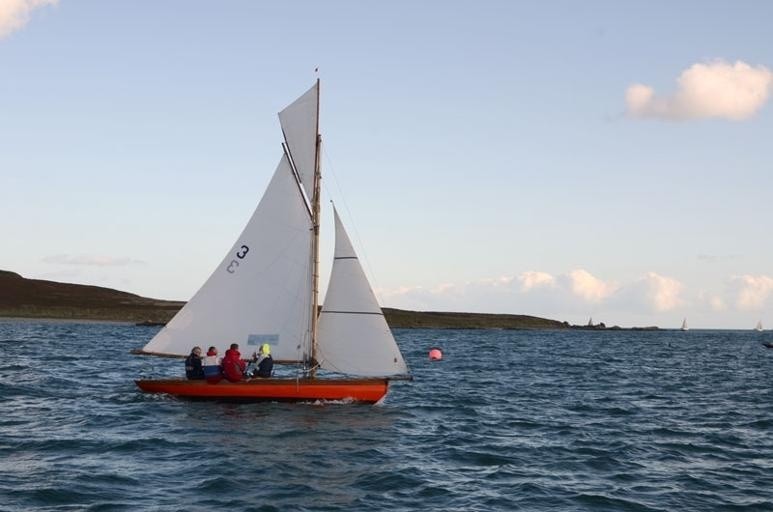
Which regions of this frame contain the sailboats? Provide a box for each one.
[122,73,413,407]
[681,319,687,331]
[588,317,593,326]
[754,320,763,331]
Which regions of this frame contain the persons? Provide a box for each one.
[184,346,205,380]
[223,344,246,383]
[244,343,274,378]
[202,347,224,384]
